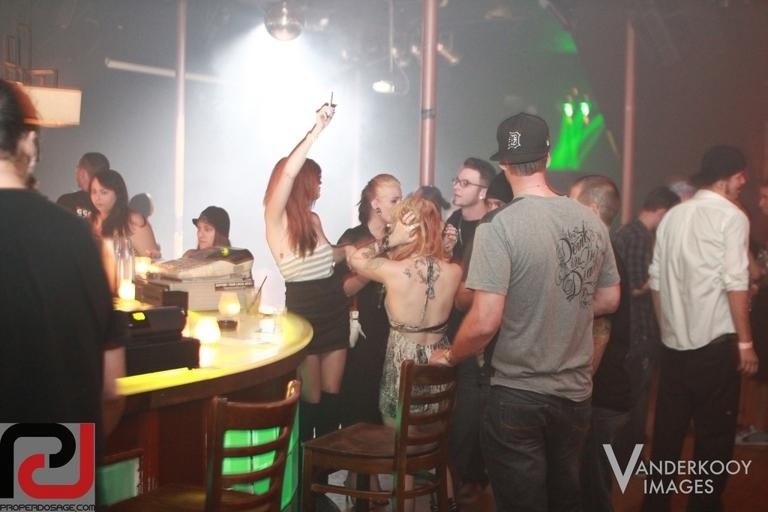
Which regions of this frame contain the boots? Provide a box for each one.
[298,390,341,495]
[430,495,459,512]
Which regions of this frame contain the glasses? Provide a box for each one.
[452,175,489,191]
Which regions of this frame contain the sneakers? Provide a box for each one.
[734,424,768,446]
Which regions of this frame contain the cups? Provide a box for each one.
[161,289,189,319]
[245,288,263,316]
[114,237,137,298]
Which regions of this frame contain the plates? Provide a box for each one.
[217,320,238,330]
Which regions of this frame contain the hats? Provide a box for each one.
[192,205,230,236]
[688,144,747,187]
[489,111,550,164]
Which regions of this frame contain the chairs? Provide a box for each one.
[107,376,305,512]
[306,359,459,511]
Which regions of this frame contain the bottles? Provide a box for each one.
[219,292,243,317]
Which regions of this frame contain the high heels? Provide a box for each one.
[344,478,390,506]
